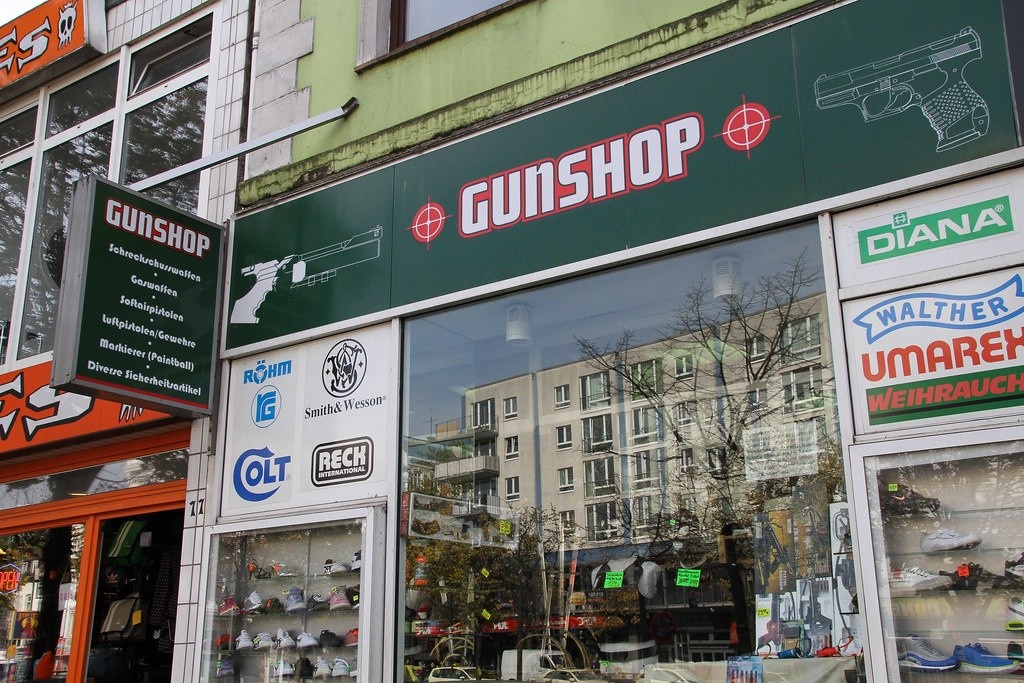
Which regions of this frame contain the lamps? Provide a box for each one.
[505,305,532,346]
[711,257,741,300]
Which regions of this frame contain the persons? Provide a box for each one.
[591,539,661,602]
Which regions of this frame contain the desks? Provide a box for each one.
[644,655,860,683]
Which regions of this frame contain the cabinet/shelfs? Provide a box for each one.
[848,424,1024,683]
[199,505,383,683]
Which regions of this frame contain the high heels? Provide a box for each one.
[244,559,300,581]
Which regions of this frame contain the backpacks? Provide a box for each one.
[88,514,156,683]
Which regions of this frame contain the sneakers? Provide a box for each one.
[219,584,360,615]
[216,654,357,679]
[218,626,358,652]
[1005,596,1024,631]
[885,482,942,515]
[888,553,1024,597]
[919,527,982,555]
[322,550,361,576]
[895,634,1024,675]
